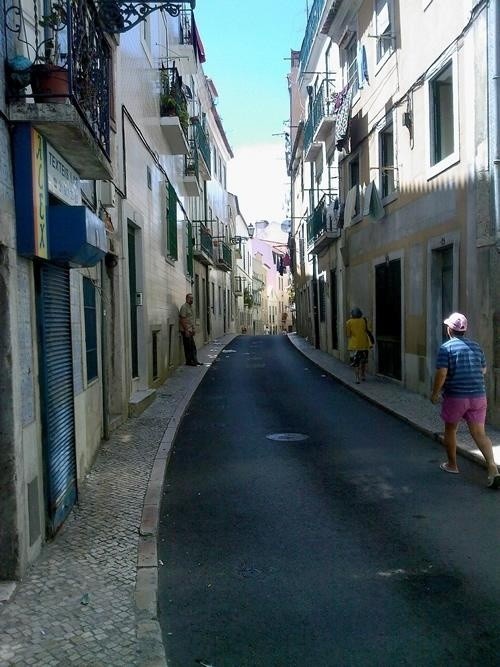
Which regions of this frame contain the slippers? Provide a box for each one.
[439,462,460,474]
[486,473,499,489]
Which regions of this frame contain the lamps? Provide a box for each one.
[240,220,256,240]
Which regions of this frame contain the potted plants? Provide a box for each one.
[161,93,189,128]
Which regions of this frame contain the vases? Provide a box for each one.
[27,63,70,103]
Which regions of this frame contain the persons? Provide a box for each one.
[346,308,372,384]
[179,294,204,366]
[430,312,500,488]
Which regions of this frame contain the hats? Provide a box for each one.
[352,308,361,316]
[443,312,468,332]
